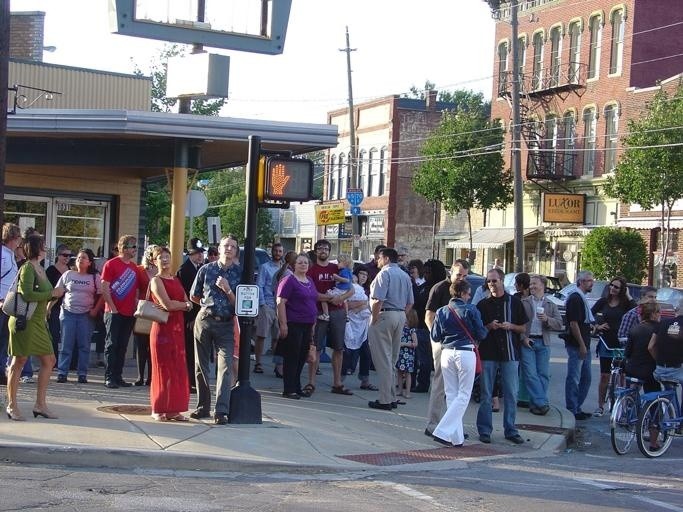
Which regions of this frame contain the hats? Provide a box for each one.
[187,238,206,255]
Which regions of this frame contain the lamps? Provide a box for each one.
[145,180,161,209]
[544,242,553,257]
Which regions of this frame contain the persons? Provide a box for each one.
[1,223,682,452]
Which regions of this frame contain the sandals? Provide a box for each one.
[283,383,315,399]
[331,384,353,395]
[254,363,263,373]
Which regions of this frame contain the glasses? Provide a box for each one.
[61,253,71,257]
[487,280,497,282]
[610,283,619,288]
[127,245,136,248]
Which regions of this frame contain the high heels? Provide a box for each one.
[33,407,58,419]
[6,406,25,421]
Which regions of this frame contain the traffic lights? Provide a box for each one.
[257,155,314,202]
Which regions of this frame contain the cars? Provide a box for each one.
[200,247,271,283]
[466,273,683,331]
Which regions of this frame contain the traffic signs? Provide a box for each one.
[347,192,364,215]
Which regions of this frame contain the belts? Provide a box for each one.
[210,315,222,321]
[451,346,471,350]
[528,335,543,338]
[381,308,406,311]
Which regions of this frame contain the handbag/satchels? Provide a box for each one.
[474,345,481,372]
[133,300,169,334]
[1,292,38,320]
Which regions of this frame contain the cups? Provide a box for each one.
[536,307,543,321]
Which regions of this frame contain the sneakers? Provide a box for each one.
[594,408,605,417]
[20,375,35,384]
[575,412,592,420]
[425,428,524,447]
[57,376,67,383]
[360,383,430,410]
[78,375,87,383]
[152,407,228,424]
[104,378,150,388]
[517,401,549,415]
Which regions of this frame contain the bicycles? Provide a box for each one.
[636,375,683,459]
[595,334,627,409]
[609,373,667,455]
